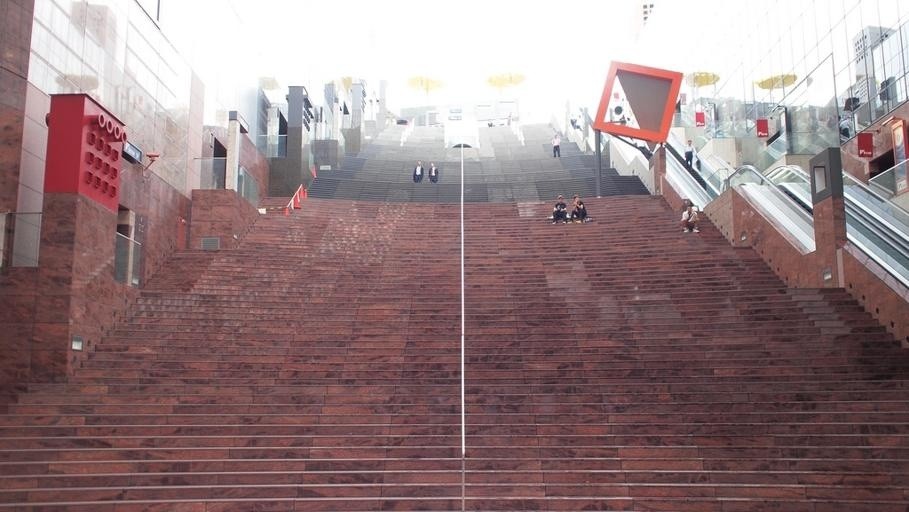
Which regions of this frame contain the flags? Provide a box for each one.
[756,119,768,137]
[695,112,705,126]
[858,133,874,158]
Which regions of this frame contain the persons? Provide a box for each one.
[680,202,700,234]
[412,158,424,184]
[553,194,568,225]
[684,139,694,169]
[551,133,561,158]
[570,194,587,224]
[569,118,583,132]
[428,162,438,183]
